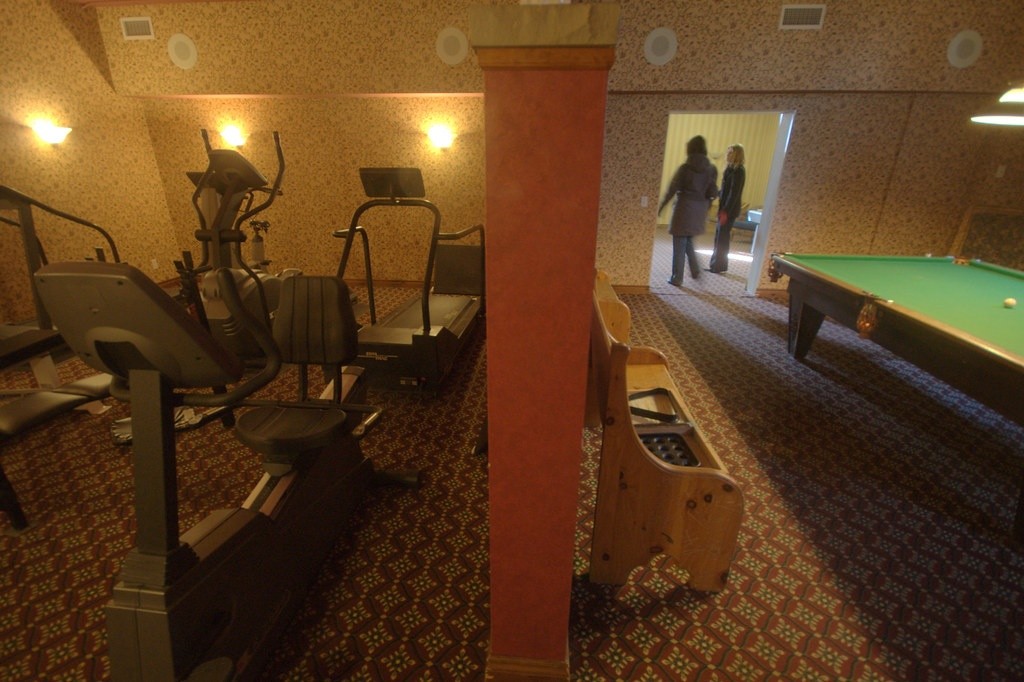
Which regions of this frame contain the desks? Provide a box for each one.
[747,210,762,254]
[766,251,1024,428]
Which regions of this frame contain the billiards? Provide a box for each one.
[862,322,872,333]
[1004,297,1017,308]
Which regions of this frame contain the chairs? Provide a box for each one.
[234,274,360,460]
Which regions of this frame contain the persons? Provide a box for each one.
[658,135,718,286]
[703,143,745,273]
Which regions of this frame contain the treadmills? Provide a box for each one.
[330,166,486,394]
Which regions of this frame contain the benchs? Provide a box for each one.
[585,267,745,594]
[711,218,757,242]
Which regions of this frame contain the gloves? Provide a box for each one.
[718,212,727,225]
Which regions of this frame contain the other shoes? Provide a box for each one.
[692,271,699,278]
[667,279,680,286]
[704,268,719,273]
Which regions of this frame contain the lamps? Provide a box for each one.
[970,81,1024,127]
